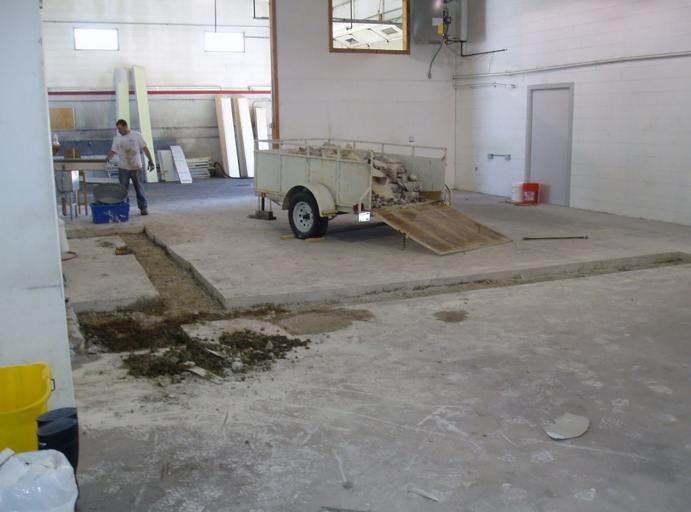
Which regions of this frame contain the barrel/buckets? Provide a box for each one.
[511,182,523,204]
[523,182,539,204]
[55,171,80,192]
[0,361,56,452]
[92,184,129,204]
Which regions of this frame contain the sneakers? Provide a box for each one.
[141,207,147,215]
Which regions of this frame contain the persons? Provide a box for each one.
[105,120,155,215]
